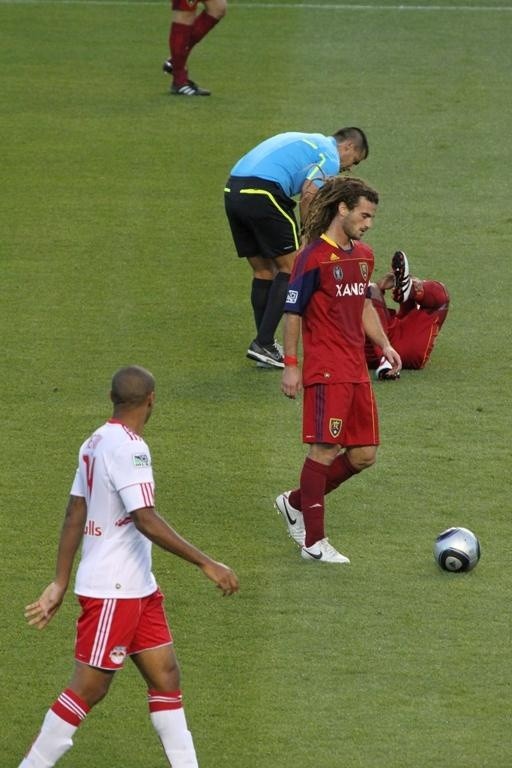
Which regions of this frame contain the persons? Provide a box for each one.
[221,124,373,373]
[162,0,230,97]
[363,247,451,384]
[269,178,406,568]
[10,358,243,768]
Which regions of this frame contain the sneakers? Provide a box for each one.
[164,57,189,76]
[257,342,284,369]
[376,356,401,381]
[247,338,286,368]
[391,250,413,304]
[274,490,308,550]
[171,80,211,96]
[303,538,351,564]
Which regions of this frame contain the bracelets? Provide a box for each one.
[282,352,300,368]
[300,222,311,235]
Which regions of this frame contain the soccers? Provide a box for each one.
[434,527,480,574]
